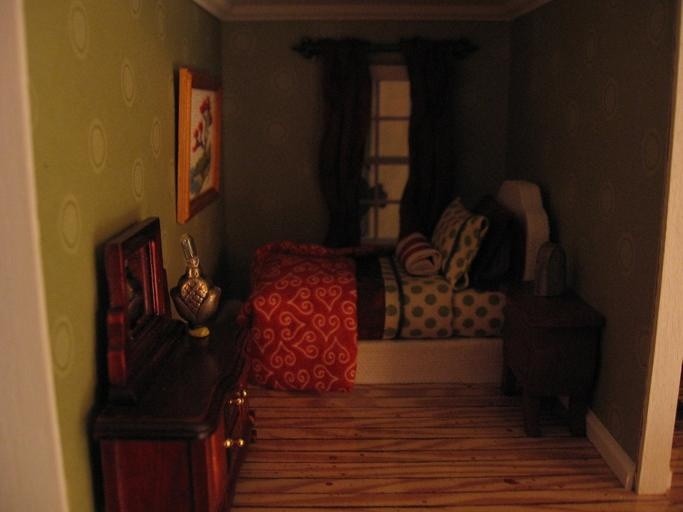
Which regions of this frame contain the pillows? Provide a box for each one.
[395,194,523,293]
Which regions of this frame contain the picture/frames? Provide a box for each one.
[175,65,224,225]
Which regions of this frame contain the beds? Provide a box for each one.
[251,176,552,389]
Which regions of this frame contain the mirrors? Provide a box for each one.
[100,215,184,405]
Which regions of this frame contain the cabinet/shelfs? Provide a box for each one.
[94,327,256,512]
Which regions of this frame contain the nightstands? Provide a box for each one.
[502,284,606,439]
